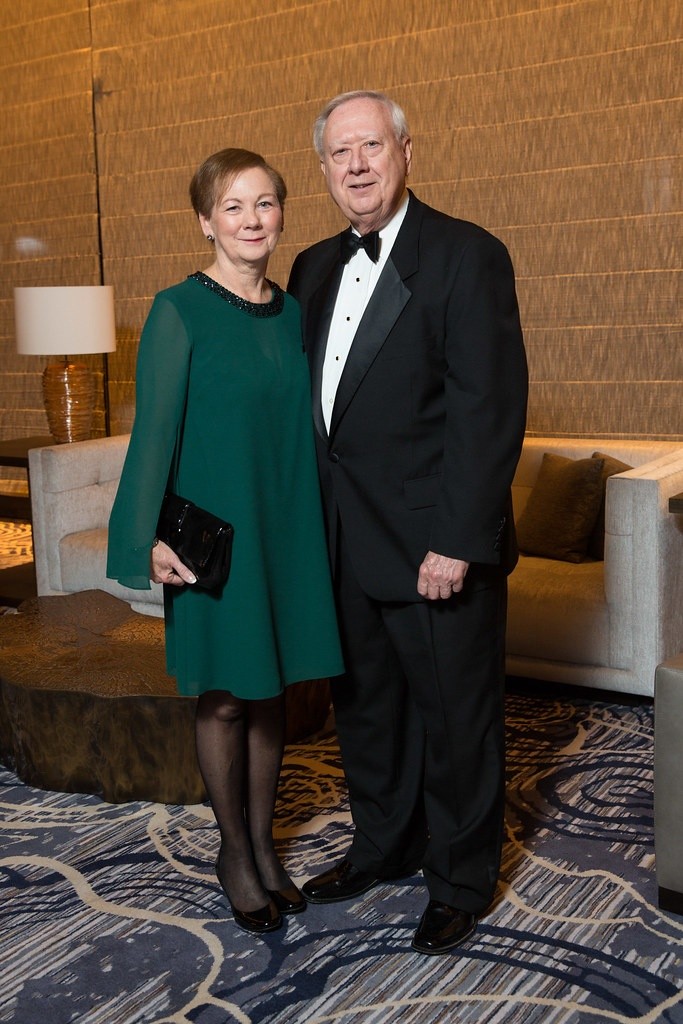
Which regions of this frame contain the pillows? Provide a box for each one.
[589,452,633,562]
[514,450,606,564]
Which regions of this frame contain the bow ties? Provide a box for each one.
[341,231,379,263]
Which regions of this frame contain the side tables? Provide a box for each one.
[0,435,58,609]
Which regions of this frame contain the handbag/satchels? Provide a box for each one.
[156,490,233,598]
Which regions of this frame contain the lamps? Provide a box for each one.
[13,285,118,444]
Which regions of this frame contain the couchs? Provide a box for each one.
[28,433,683,708]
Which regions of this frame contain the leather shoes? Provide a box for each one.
[411,900,476,954]
[301,860,418,903]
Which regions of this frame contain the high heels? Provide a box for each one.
[257,864,306,914]
[215,845,283,932]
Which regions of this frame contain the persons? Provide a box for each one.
[105,148,345,931]
[286,91,529,955]
